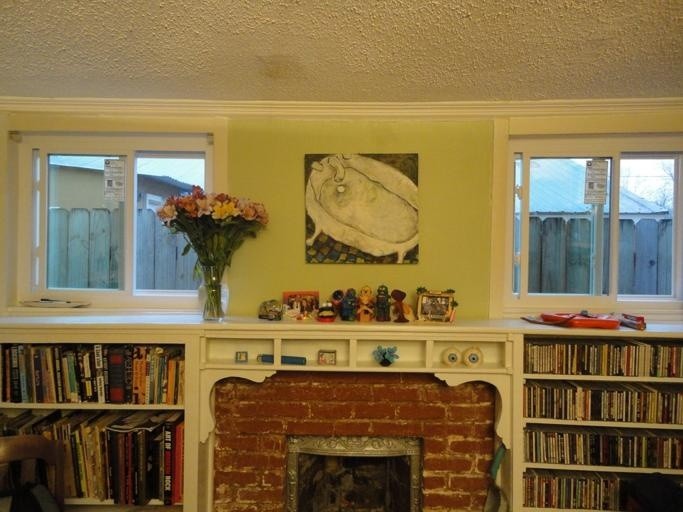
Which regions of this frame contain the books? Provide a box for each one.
[524,340,683,509]
[1,343,186,404]
[0,412,184,506]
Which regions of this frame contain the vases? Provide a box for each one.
[202,263,224,320]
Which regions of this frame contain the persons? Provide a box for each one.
[290,294,311,314]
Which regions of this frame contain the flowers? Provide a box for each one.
[156,184,269,318]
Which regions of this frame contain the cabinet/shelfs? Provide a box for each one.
[200,327,512,375]
[512,335,683,512]
[0,327,201,511]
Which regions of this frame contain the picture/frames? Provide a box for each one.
[418,293,453,321]
[282,290,320,319]
